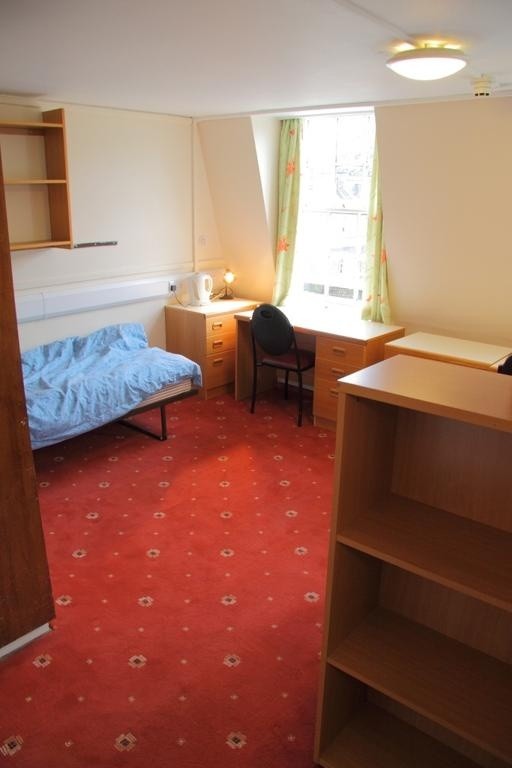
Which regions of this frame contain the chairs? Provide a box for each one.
[250,304,314,427]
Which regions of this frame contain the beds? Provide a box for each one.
[20,322,202,451]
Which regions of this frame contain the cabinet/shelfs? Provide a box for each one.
[0,107,74,251]
[313,354,512,768]
[166,296,263,400]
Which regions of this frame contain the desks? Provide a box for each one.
[234,313,404,434]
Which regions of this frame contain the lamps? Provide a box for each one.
[385,38,468,82]
[218,269,235,299]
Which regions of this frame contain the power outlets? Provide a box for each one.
[169,281,177,293]
